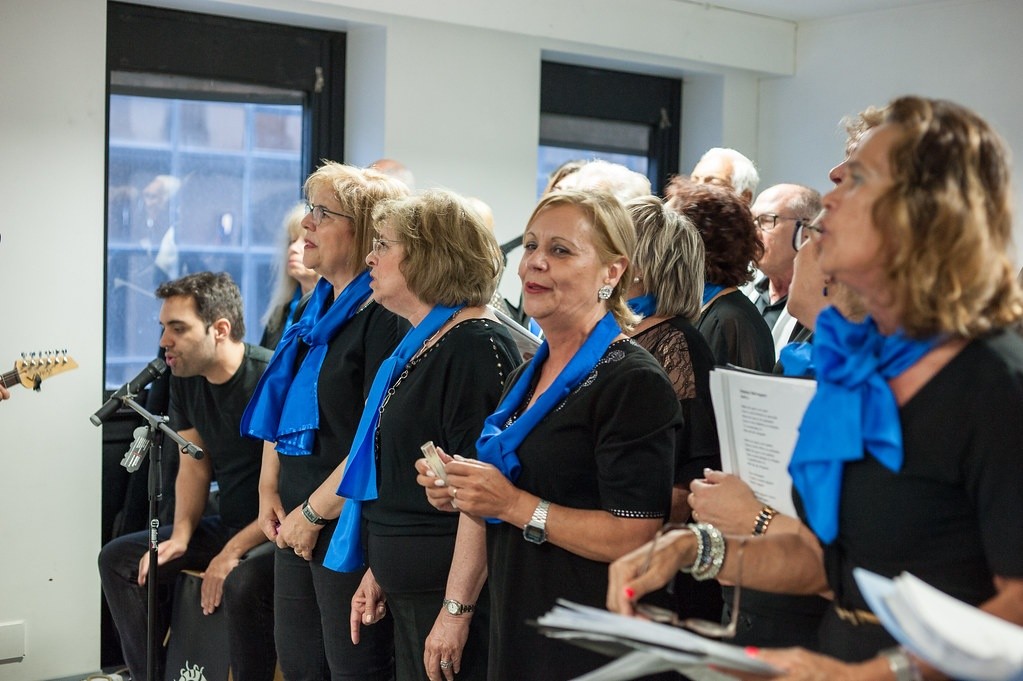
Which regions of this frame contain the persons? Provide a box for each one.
[98,97,1023,681]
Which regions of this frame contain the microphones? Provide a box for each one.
[90,358,167,427]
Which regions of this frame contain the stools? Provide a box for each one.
[165,568,285,681]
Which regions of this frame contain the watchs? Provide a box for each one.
[523,499,551,545]
[301,499,333,523]
[442,598,477,615]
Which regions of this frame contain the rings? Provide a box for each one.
[440,661,452,669]
[691,511,697,521]
[452,487,458,500]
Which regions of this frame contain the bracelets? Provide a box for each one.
[680,522,726,582]
[881,647,919,681]
[752,506,780,537]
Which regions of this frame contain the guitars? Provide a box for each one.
[1,347,80,394]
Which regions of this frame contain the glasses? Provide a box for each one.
[752,214,810,230]
[636,523,748,638]
[792,221,822,252]
[372,237,402,255]
[305,204,354,226]
